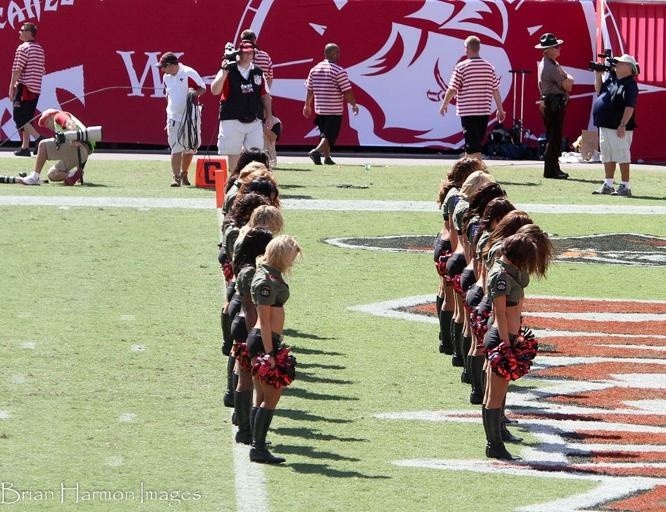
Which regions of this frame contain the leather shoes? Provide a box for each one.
[545,171,567,179]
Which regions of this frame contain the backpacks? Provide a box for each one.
[487,123,512,160]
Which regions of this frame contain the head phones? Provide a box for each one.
[238,40,258,60]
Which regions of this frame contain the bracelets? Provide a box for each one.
[351,102,356,107]
[619,122,627,128]
[220,67,227,72]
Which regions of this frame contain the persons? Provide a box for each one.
[153,48,208,191]
[298,40,361,167]
[7,20,47,157]
[236,28,276,94]
[17,106,96,188]
[214,143,305,466]
[438,34,509,160]
[428,150,555,466]
[590,53,638,199]
[201,38,274,177]
[534,31,575,182]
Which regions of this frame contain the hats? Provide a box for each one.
[614,53,640,75]
[535,33,564,49]
[240,40,254,52]
[154,52,178,68]
[37,109,60,127]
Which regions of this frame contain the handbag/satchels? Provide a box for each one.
[501,143,529,159]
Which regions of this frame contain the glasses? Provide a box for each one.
[244,51,255,55]
[19,29,28,32]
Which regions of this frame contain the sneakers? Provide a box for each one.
[179,171,190,185]
[170,174,181,187]
[592,182,616,195]
[324,159,335,165]
[20,174,40,186]
[308,148,322,165]
[64,169,84,185]
[14,148,31,157]
[611,183,632,198]
[33,136,45,154]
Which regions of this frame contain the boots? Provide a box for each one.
[502,398,518,425]
[502,419,524,443]
[250,407,286,464]
[435,295,487,405]
[485,407,523,461]
[221,306,255,445]
